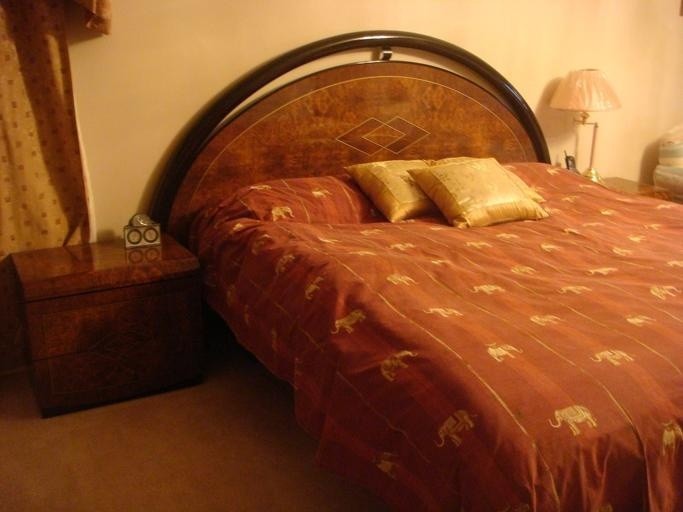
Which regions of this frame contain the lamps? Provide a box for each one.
[549,68,623,187]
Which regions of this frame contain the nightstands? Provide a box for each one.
[10,231,209,420]
[601,177,673,202]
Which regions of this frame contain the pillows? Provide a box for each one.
[208,176,382,226]
[405,158,549,230]
[429,154,544,224]
[345,156,434,222]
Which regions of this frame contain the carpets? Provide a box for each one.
[0,370,392,511]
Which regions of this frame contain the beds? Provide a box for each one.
[149,31,682,511]
